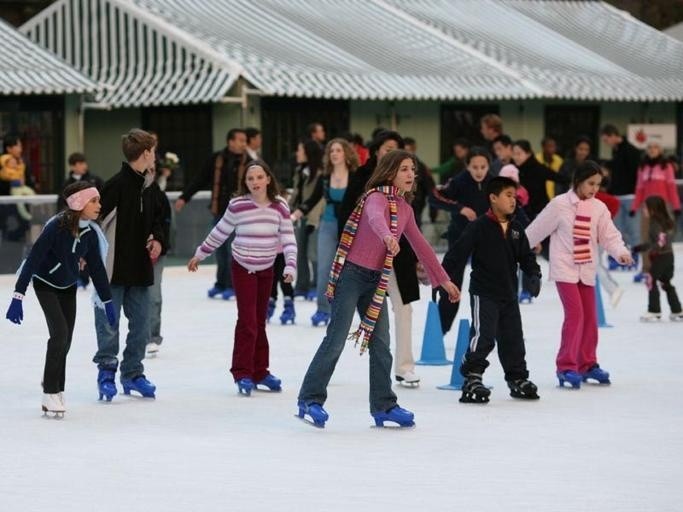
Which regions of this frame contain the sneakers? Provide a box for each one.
[41,393,66,414]
[119,371,158,394]
[296,399,328,424]
[147,342,159,352]
[371,405,415,424]
[607,253,682,321]
[207,284,332,392]
[458,364,611,399]
[96,366,117,396]
[393,370,422,384]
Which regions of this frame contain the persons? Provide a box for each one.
[523,157,637,390]
[77,128,171,401]
[296,148,461,429]
[174,112,682,322]
[382,257,426,391]
[5,181,117,419]
[0,136,108,211]
[434,177,541,404]
[186,160,298,398]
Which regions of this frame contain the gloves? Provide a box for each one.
[5,292,25,326]
[103,299,117,330]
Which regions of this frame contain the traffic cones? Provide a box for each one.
[437,318,492,392]
[594,272,612,328]
[416,301,453,366]
